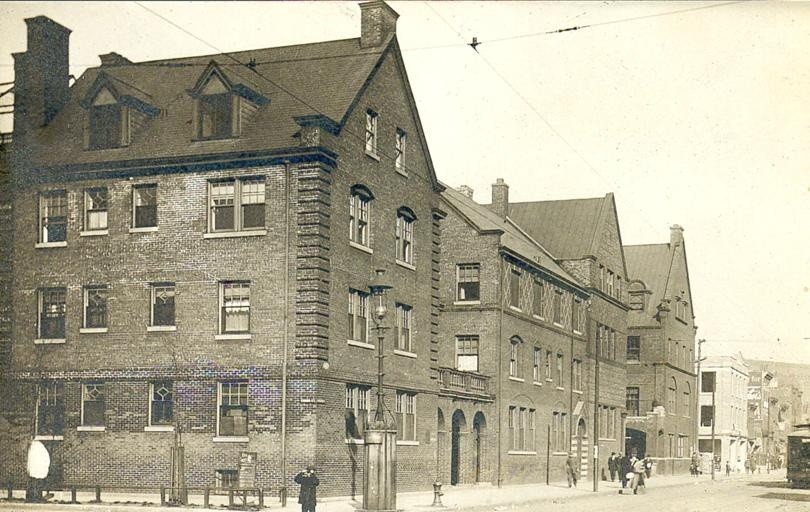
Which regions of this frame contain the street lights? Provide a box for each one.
[367,268,396,428]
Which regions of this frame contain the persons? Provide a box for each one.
[691,451,756,476]
[608,453,653,488]
[294,467,319,511]
